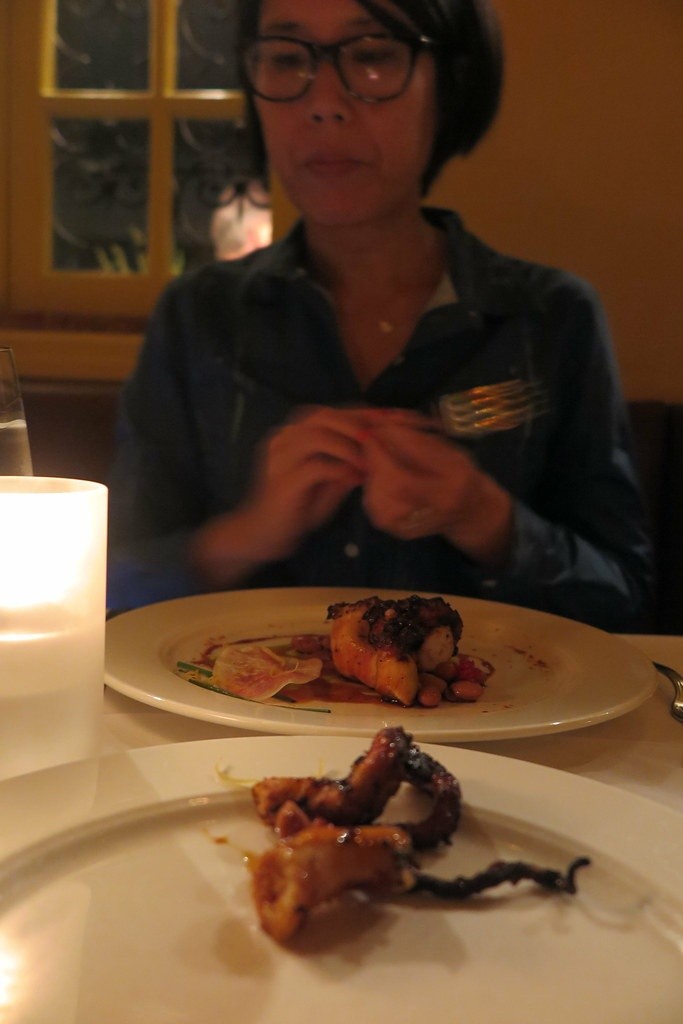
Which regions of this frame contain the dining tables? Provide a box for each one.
[106,635,683,811]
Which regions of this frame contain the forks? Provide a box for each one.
[425,380,548,438]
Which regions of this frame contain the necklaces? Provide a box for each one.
[308,229,441,334]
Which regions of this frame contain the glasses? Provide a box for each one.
[236,32,434,104]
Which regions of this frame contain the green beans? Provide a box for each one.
[419,661,483,706]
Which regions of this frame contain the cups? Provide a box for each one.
[0,346,35,476]
[0,476,108,699]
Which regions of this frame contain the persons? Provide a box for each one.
[107,0,670,635]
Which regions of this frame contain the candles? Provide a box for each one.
[0,477,108,776]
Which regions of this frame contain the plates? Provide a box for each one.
[0,737,683,1024]
[106,586,658,735]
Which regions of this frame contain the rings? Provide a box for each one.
[402,500,430,526]
